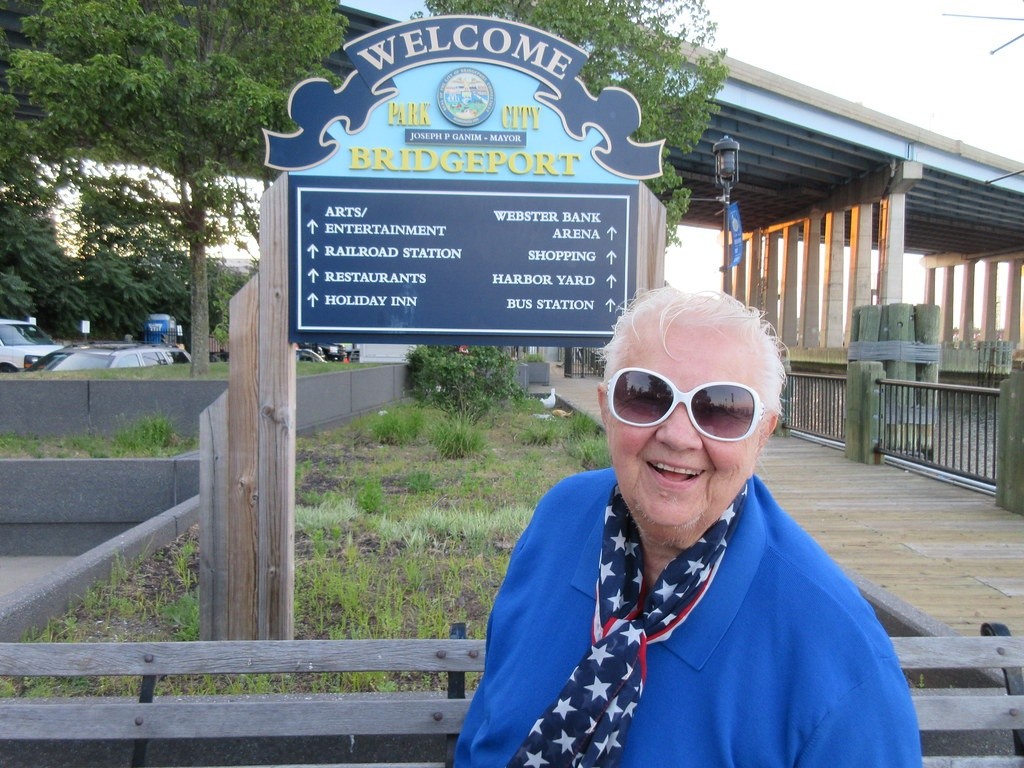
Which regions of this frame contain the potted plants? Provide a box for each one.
[525,353,550,383]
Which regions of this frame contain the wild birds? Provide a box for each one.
[540,388,574,417]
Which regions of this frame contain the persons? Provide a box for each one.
[453,287,924,768]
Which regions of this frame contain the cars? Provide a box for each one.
[0,318,67,373]
[19,339,192,372]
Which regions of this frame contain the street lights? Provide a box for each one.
[710,134,741,295]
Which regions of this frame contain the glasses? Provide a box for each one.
[608,367,769,442]
